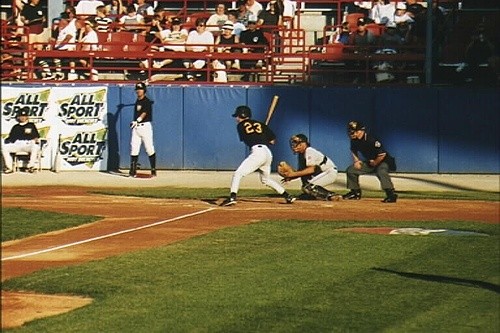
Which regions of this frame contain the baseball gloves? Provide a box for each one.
[276,160,296,179]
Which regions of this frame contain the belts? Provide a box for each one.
[137,124,144,126]
[258,146,262,148]
[334,168,337,169]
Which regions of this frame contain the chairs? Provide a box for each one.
[15,137,48,172]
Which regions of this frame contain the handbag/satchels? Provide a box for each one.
[385,157,396,171]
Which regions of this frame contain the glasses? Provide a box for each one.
[196,24,204,27]
[248,22,255,24]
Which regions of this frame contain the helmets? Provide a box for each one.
[232,106,251,118]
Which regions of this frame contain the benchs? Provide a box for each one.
[0,0,500,83]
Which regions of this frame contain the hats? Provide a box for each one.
[172,17,181,25]
[222,15,258,30]
[357,2,406,27]
[295,134,310,147]
[347,120,360,135]
[135,82,145,90]
[19,109,28,116]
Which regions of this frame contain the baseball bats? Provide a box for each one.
[53,133,63,173]
[264,95,279,126]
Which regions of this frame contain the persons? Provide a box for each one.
[342,122,396,203]
[0,0,500,177]
[220,107,296,206]
[278,134,343,201]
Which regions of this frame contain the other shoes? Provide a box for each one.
[151,170,156,177]
[231,61,262,68]
[343,191,361,200]
[384,194,397,202]
[221,197,237,205]
[42,72,99,82]
[129,173,136,178]
[299,194,316,200]
[5,169,12,173]
[29,167,34,173]
[329,194,343,201]
[286,195,296,204]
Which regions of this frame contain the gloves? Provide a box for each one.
[130,120,139,129]
